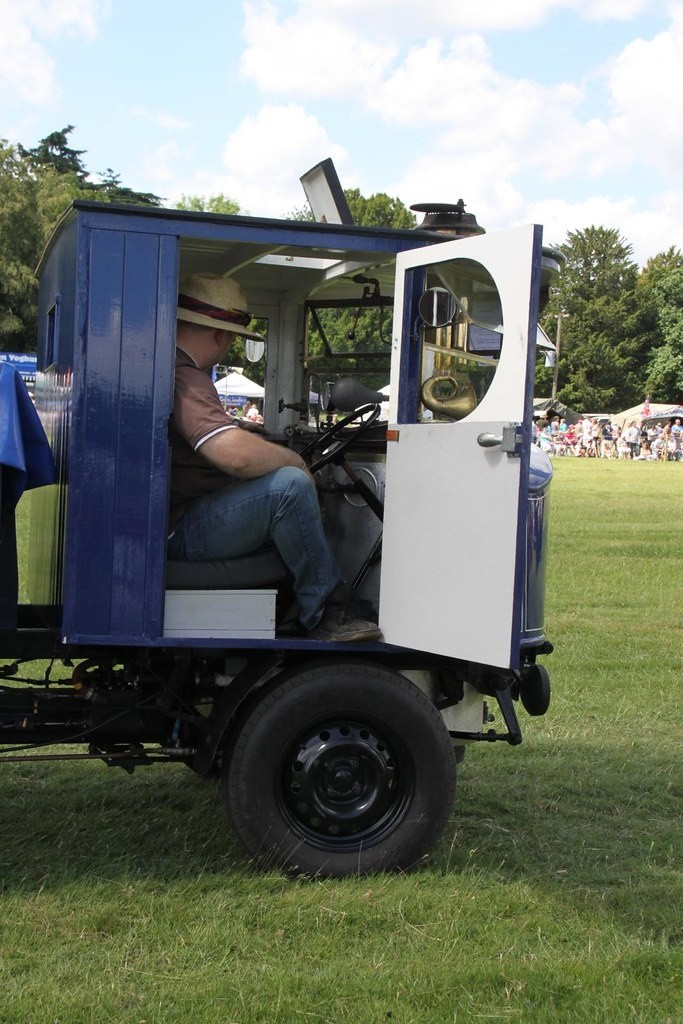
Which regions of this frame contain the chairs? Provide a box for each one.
[160,547,288,587]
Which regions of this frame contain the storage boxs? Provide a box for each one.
[165,588,279,639]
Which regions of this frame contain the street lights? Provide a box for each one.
[550,308,571,400]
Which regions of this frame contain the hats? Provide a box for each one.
[177,271,265,342]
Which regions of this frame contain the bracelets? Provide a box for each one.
[301,462,305,471]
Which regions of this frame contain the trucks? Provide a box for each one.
[0,157,566,882]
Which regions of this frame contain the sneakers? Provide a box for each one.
[315,604,382,642]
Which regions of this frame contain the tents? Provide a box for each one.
[610,402,683,435]
[532,398,585,426]
[213,372,265,416]
[355,385,433,422]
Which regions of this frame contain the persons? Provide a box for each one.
[166,273,382,644]
[225,401,263,424]
[530,416,683,463]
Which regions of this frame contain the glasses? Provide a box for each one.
[231,306,255,327]
